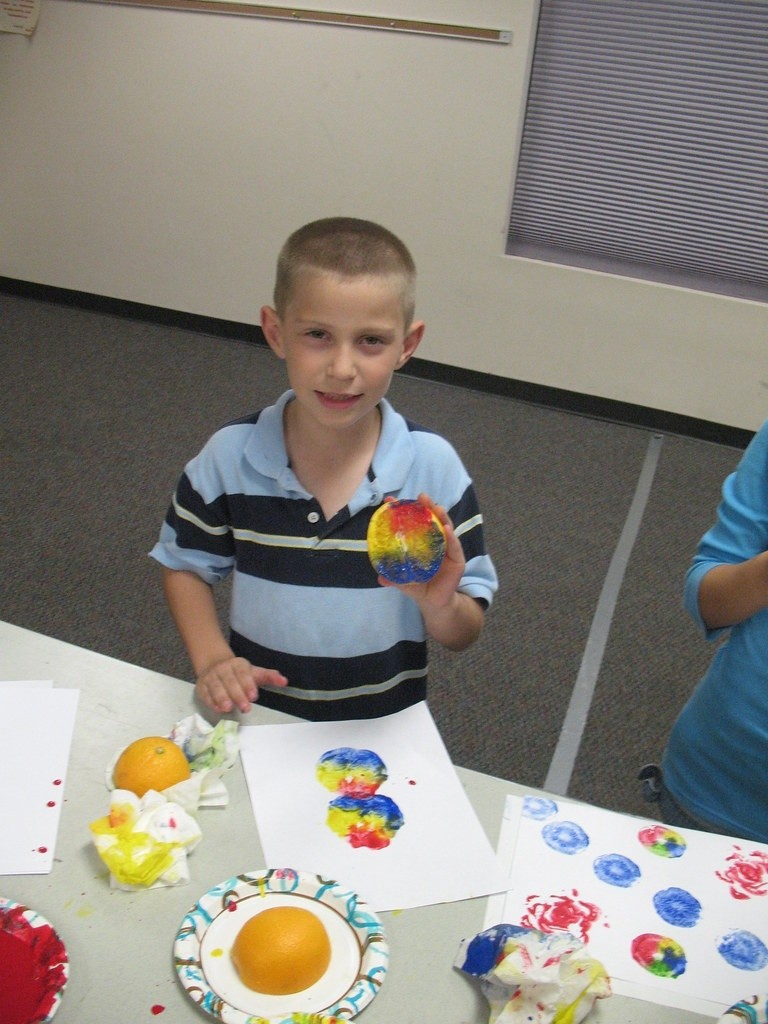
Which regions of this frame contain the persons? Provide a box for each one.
[148,216,499,726]
[634,420,768,844]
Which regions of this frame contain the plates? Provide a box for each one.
[0,896,68,1024]
[173,865,390,1024]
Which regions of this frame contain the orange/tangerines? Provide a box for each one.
[366,498,446,585]
[111,736,189,797]
[230,905,331,995]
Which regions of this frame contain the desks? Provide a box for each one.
[0,623,768,1024]
